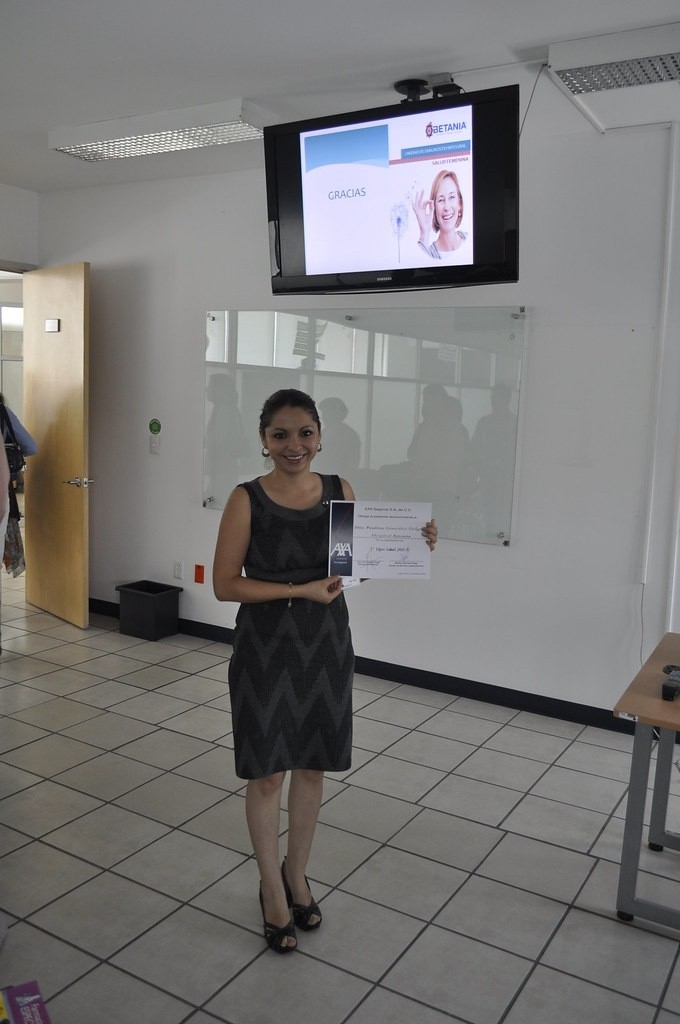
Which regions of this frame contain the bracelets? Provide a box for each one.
[288,582,292,608]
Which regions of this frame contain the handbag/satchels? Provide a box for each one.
[5,446,24,473]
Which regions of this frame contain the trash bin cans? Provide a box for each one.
[116,579,184,642]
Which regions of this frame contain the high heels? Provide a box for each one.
[282,856,323,931]
[259,880,298,953]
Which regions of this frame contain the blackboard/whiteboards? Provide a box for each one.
[199,304,528,548]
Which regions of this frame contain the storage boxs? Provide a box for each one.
[115,579,184,641]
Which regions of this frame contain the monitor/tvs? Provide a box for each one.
[263,85,521,296]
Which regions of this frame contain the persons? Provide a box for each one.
[213,388,437,954]
[0,393,37,657]
[411,170,471,259]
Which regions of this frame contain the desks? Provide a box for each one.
[613,632,680,931]
